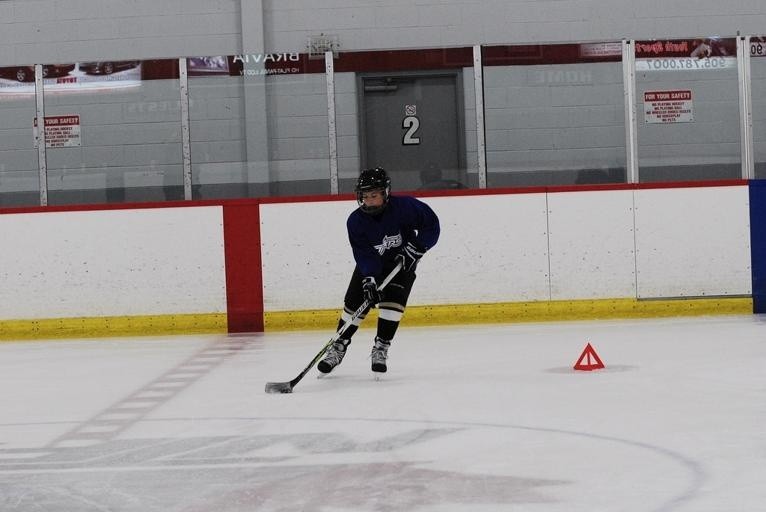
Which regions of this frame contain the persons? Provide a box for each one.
[315,166,442,374]
[416,167,472,192]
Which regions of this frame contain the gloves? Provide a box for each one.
[362,276,384,309]
[394,242,425,273]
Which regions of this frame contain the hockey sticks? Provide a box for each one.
[265,261,403,393]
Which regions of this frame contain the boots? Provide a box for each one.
[372,336,390,373]
[318,338,351,373]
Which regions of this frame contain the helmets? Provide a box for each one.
[419,167,443,185]
[353,167,391,214]
